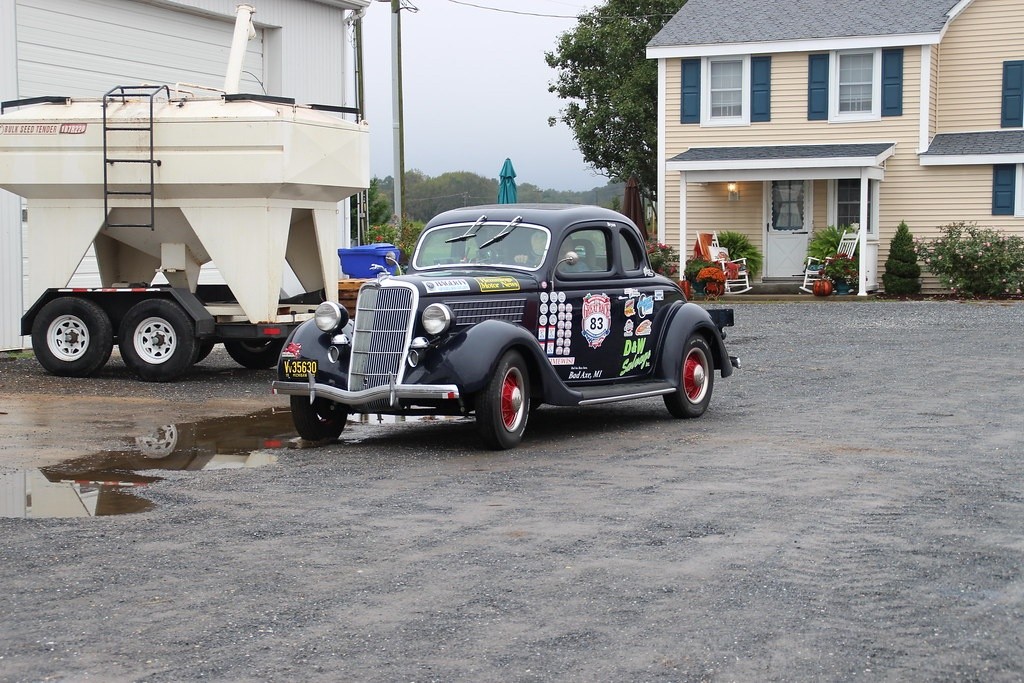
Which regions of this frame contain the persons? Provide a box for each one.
[515,236,589,271]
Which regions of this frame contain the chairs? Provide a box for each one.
[572,238,598,270]
[695,230,753,294]
[799,229,860,294]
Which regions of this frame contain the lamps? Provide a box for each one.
[727,181,737,192]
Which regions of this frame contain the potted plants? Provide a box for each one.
[685,255,717,292]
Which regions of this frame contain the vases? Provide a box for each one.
[812,281,832,296]
[703,279,720,298]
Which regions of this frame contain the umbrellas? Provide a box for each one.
[497,158,518,203]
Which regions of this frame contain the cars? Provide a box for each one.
[273,203,741,451]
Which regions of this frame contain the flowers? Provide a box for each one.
[696,267,726,285]
[818,253,859,291]
[644,237,680,277]
